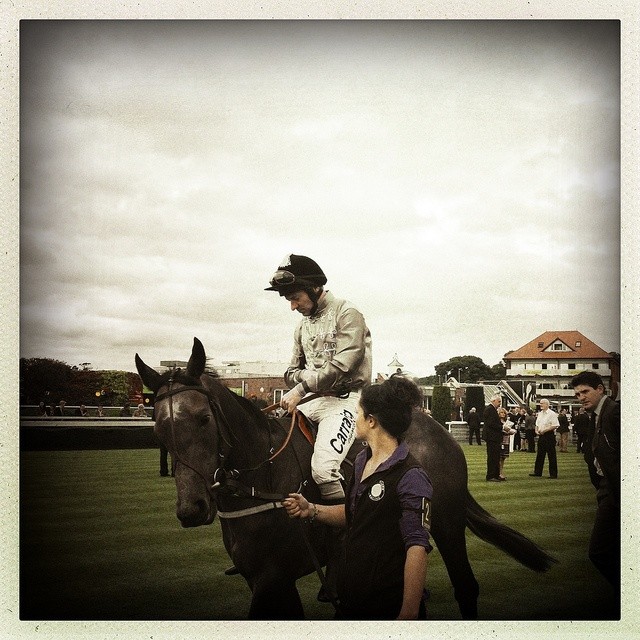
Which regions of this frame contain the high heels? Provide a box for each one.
[523,449,527,452]
[519,449,523,451]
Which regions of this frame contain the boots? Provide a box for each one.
[225,566,241,575]
[318,497,349,603]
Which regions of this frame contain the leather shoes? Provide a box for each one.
[547,477,557,479]
[529,473,542,476]
[497,476,505,481]
[487,478,500,482]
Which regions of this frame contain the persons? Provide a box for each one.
[120,402,132,417]
[134,404,147,417]
[49,403,56,416]
[482,395,505,482]
[466,407,481,445]
[529,398,561,479]
[34,401,50,416]
[571,370,620,594]
[263,254,372,536]
[557,408,589,453]
[54,401,66,416]
[498,407,535,478]
[281,370,434,620]
[76,404,89,417]
[94,404,106,416]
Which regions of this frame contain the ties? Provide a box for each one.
[588,412,598,490]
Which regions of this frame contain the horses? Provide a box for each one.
[134,335,560,621]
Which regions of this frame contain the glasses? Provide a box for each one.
[269,270,325,287]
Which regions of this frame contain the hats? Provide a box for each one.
[264,255,327,291]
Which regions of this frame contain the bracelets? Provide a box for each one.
[305,504,318,524]
